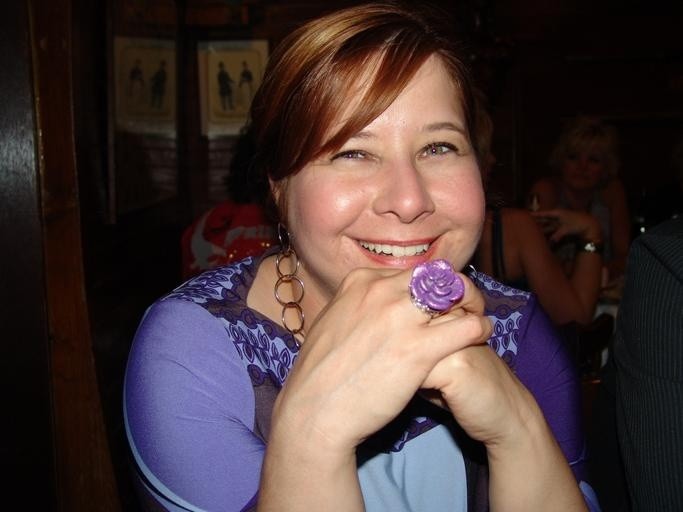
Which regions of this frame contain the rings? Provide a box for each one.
[408,258,465,318]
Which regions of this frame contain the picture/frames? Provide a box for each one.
[113,38,179,134]
[196,39,269,138]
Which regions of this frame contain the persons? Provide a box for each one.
[130,54,170,111]
[124,6,602,512]
[613,214,682,512]
[468,91,606,332]
[217,58,253,111]
[528,112,632,306]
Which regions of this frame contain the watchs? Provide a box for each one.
[574,239,605,255]
